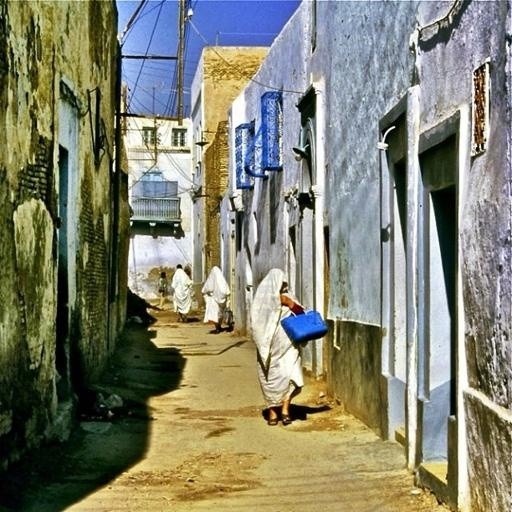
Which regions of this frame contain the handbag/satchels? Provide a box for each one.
[281,310,328,344]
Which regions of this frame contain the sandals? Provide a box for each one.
[281,413,291,425]
[268,417,278,425]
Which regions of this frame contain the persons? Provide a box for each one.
[251,268,306,425]
[159,271,168,307]
[201,265,231,333]
[171,264,193,322]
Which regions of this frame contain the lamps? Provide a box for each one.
[377,126,397,151]
[294,144,310,161]
